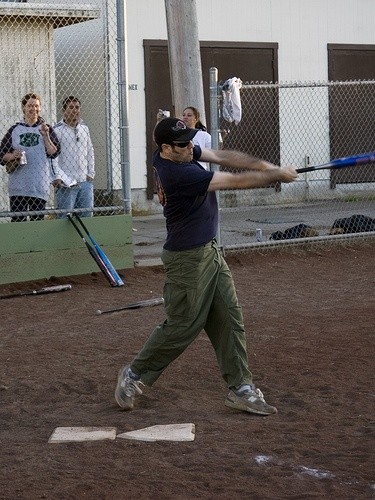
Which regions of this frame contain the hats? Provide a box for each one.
[154,117,199,144]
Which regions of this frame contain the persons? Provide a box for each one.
[157,106,212,172]
[48,96,95,218]
[114,117,299,415]
[0,94,61,223]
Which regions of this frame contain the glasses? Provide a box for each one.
[173,139,192,148]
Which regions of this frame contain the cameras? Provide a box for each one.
[162,111,170,119]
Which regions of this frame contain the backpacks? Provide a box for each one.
[328,215,375,235]
[269,224,319,241]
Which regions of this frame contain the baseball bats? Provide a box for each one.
[96,298,164,316]
[297,152,375,173]
[66,212,124,287]
[0,284,72,297]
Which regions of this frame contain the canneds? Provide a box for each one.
[20,150,28,165]
[256,229,263,242]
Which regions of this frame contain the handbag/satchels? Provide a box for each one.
[6,147,25,175]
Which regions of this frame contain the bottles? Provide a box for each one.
[19,150,27,165]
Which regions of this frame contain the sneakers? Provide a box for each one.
[115,365,145,410]
[225,387,277,416]
